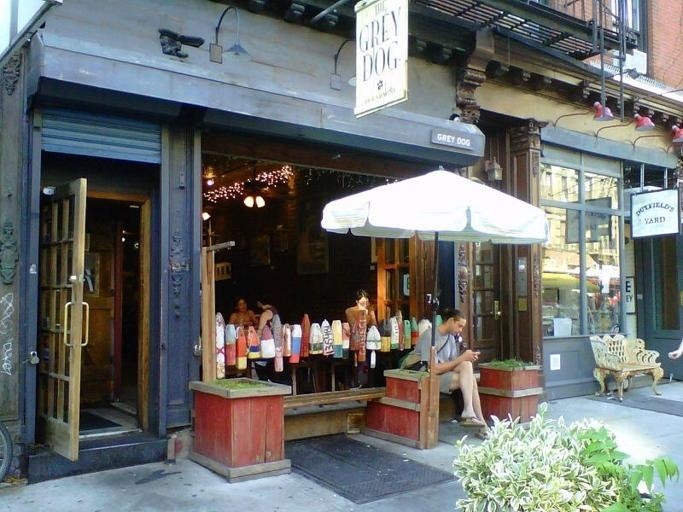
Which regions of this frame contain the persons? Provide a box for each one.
[345,288,378,331]
[254,293,279,338]
[667,338,683,360]
[588,292,618,335]
[228,296,256,329]
[405,308,492,441]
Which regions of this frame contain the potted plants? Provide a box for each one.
[480,358,545,428]
[188,373,294,481]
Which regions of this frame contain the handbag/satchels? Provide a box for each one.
[401,353,426,371]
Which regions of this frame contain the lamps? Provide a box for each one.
[550,98,683,151]
[329,38,358,94]
[483,155,504,182]
[208,4,254,67]
[240,158,268,209]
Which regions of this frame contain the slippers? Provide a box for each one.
[461,417,491,440]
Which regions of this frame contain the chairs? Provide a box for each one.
[590,329,666,402]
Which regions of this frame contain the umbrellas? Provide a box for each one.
[319,169,551,344]
